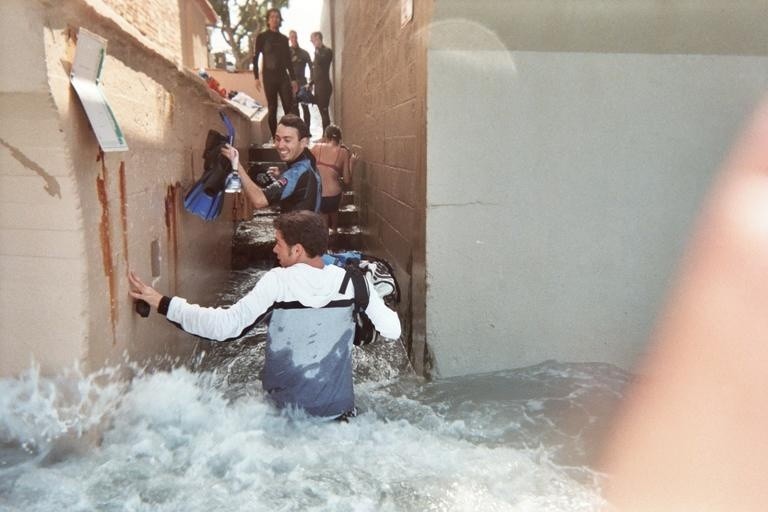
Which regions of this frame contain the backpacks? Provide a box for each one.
[324,249,401,347]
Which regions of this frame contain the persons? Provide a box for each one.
[286,25,313,129]
[127,209,402,424]
[595,86,768,512]
[304,32,333,131]
[221,110,323,268]
[308,124,360,243]
[250,8,299,143]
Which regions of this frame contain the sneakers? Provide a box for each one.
[225,171,242,194]
